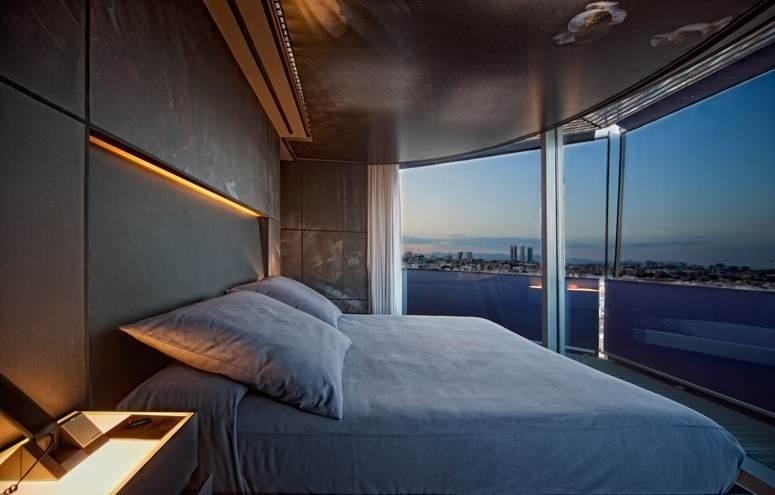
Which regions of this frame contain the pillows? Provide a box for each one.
[118,275,352,423]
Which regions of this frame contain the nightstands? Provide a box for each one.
[0,409,215,493]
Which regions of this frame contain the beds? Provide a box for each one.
[123,315,744,493]
